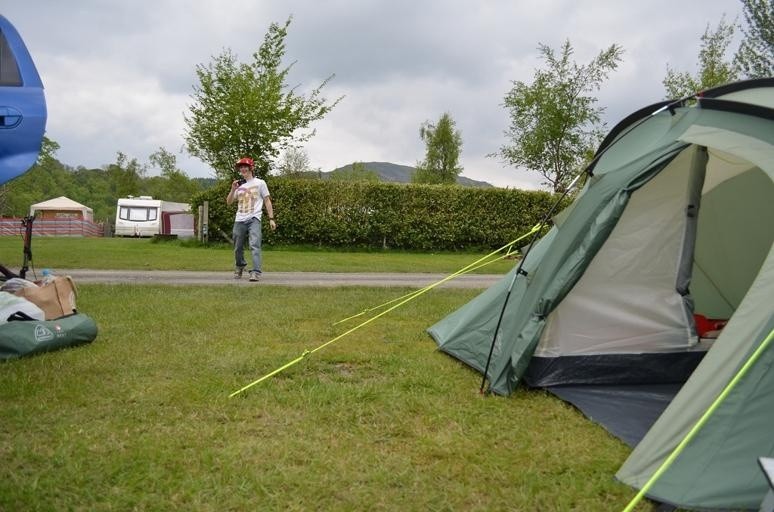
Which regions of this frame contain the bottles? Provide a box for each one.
[41,270,56,287]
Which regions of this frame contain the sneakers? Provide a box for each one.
[248,271,263,281]
[234,266,244,279]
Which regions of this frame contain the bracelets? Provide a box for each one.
[268,218,274,221]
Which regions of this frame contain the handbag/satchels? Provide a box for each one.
[0,277,98,363]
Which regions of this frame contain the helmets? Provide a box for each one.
[235,158,255,167]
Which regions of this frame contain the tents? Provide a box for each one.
[424,80,773,511]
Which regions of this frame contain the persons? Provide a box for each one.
[225,158,276,282]
